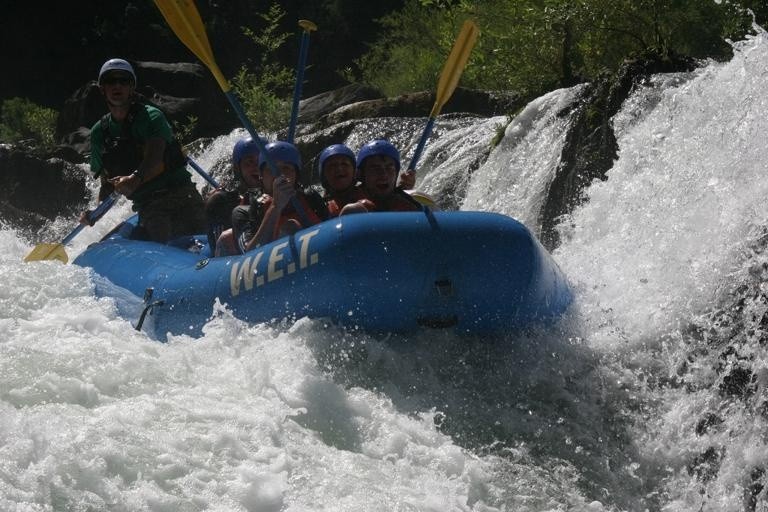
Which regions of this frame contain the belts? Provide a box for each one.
[138,188,180,200]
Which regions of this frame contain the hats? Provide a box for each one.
[98,57,137,91]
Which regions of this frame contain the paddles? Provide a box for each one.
[155,0,313,224]
[24,191,118,263]
[408,20,480,170]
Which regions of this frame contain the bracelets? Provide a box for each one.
[131,170,146,185]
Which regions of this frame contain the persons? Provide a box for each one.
[76,57,208,247]
[230,143,327,250]
[310,144,415,199]
[320,138,423,221]
[203,135,271,259]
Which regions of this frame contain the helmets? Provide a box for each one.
[356,139,400,179]
[232,135,270,166]
[257,141,303,175]
[318,143,357,182]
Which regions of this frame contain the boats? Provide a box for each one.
[73,189,579,343]
